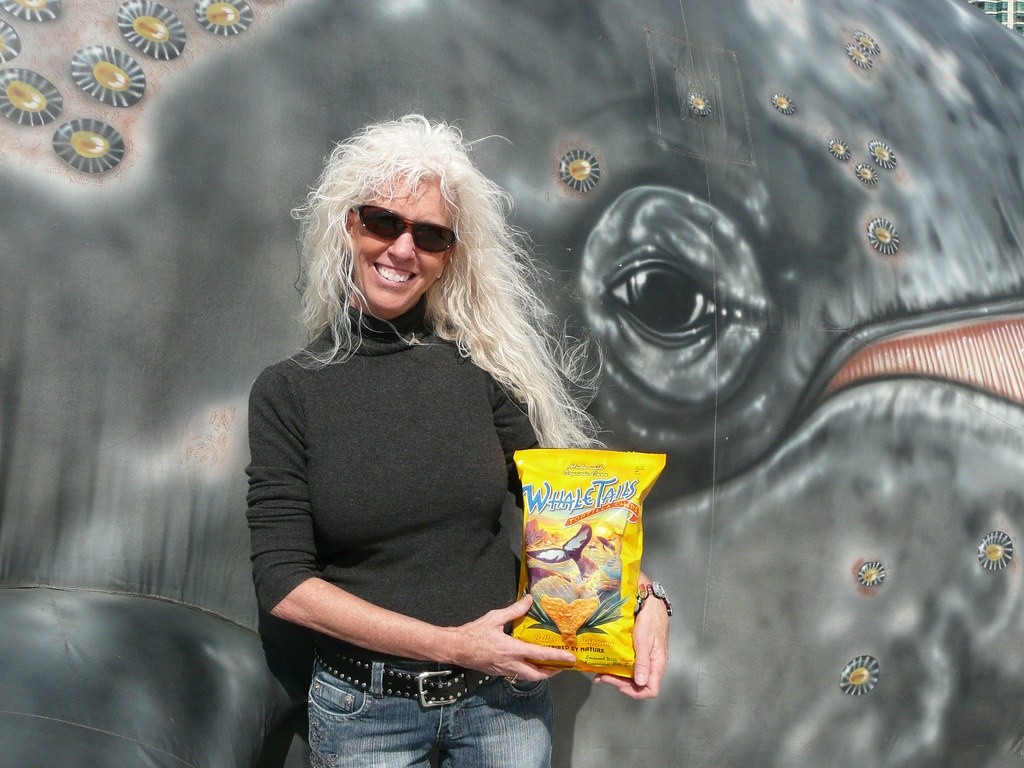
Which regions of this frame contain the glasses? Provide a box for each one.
[353,205,457,255]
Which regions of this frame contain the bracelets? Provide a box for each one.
[634,580,673,618]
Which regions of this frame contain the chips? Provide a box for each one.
[542,595,598,639]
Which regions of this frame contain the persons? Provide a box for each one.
[248,113,674,768]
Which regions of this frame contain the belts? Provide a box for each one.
[309,635,500,707]
